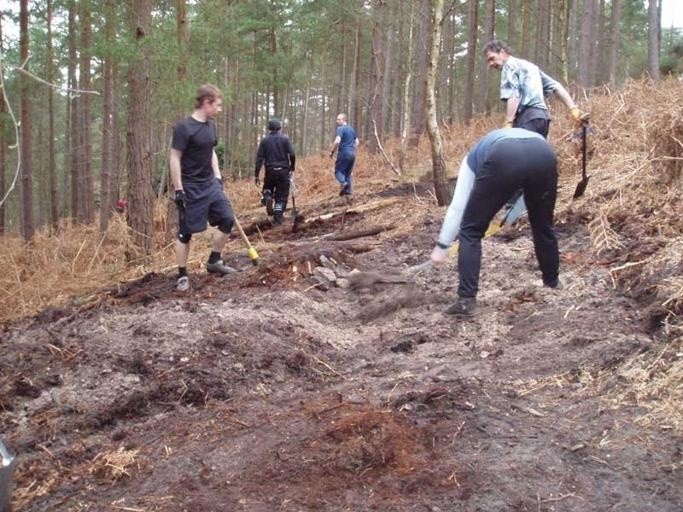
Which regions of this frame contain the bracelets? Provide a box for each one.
[330,149,334,153]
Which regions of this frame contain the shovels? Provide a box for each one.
[573,112,591,198]
[288,196,296,233]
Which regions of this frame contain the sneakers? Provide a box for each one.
[338,182,352,196]
[542,279,565,292]
[439,296,481,318]
[204,259,236,275]
[176,275,190,292]
[264,197,285,225]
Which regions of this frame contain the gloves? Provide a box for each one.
[502,121,513,129]
[215,177,224,192]
[568,104,587,126]
[172,189,190,210]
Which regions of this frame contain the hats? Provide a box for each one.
[267,119,282,132]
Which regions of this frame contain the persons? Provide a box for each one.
[481,38,589,238]
[428,125,563,318]
[254,117,296,224]
[169,83,239,294]
[328,112,360,201]
